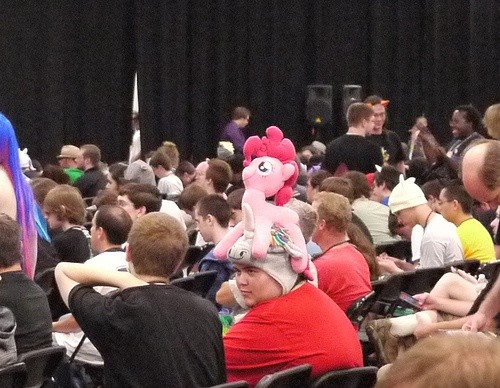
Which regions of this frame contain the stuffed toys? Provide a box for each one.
[213,126,314,281]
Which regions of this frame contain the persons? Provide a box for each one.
[0,95,500,388]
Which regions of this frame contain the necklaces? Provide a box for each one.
[423,210,434,229]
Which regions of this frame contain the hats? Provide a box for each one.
[387,174,428,215]
[55,144,81,159]
[226,202,304,297]
[123,159,156,186]
[18,147,30,168]
[311,140,327,154]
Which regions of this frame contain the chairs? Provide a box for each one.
[0,241,500,388]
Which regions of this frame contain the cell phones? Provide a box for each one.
[398,291,423,310]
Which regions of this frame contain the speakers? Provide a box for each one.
[342,85,362,122]
[305,84,332,126]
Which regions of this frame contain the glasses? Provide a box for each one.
[448,118,466,128]
[115,199,144,207]
[42,209,63,219]
